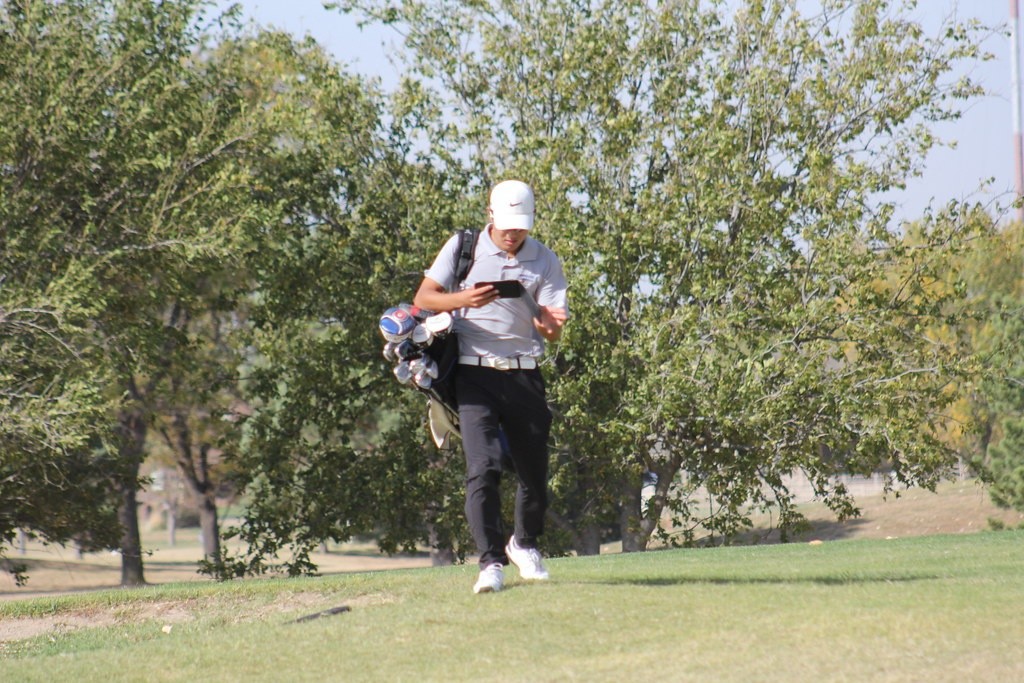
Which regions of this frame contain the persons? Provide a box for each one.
[412,180,568,594]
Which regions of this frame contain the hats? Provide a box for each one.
[489,179,535,231]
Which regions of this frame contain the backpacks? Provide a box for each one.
[395,228,482,396]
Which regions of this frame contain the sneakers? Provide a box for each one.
[472,562,504,594]
[504,534,549,581]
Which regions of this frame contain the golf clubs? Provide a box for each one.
[383,339,439,390]
